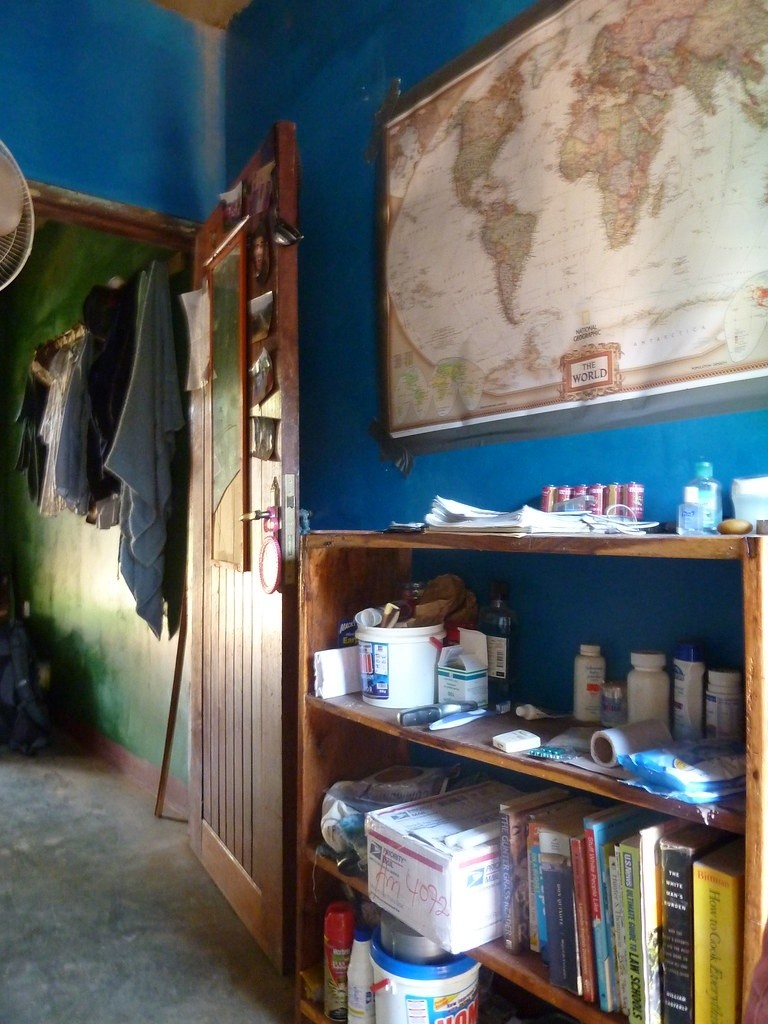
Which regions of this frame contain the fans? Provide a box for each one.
[0,138,36,290]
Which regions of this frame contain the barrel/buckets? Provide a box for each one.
[369,925,483,1023]
[354,624,446,709]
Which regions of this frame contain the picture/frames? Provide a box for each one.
[368,0,768,476]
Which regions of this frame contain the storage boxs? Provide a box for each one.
[365,779,527,953]
[435,627,490,709]
[366,763,444,786]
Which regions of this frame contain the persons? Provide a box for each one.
[249,229,268,284]
[509,812,531,864]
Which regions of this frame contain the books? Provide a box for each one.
[498,788,744,1024]
[425,495,593,534]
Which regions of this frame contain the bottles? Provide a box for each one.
[706,668,742,739]
[678,488,703,535]
[627,653,669,724]
[575,644,605,722]
[684,463,722,531]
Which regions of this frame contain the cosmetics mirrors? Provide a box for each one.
[258,507,281,594]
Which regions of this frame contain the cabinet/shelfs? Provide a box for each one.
[293,531,768,1024]
